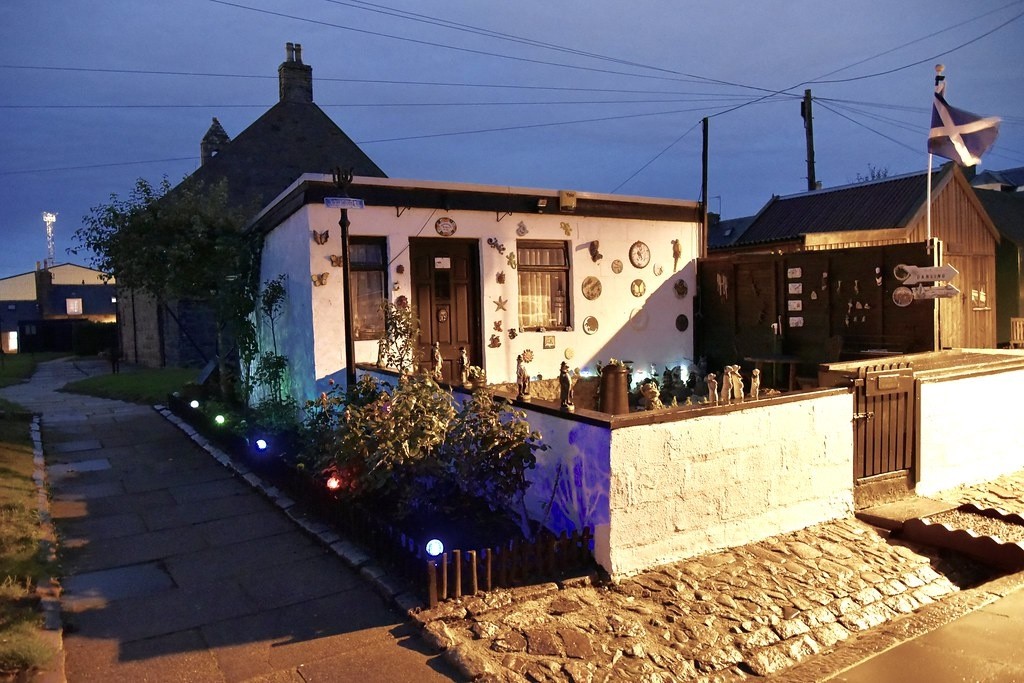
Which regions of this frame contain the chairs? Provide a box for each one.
[794,334,843,389]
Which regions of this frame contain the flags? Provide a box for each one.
[927,77,999,170]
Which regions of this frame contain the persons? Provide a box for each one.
[517,355,530,396]
[707,372,720,406]
[658,395,692,409]
[749,368,761,400]
[459,347,471,383]
[431,342,444,379]
[559,361,574,407]
[720,366,733,405]
[731,364,745,404]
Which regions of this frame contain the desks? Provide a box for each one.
[743,352,802,392]
